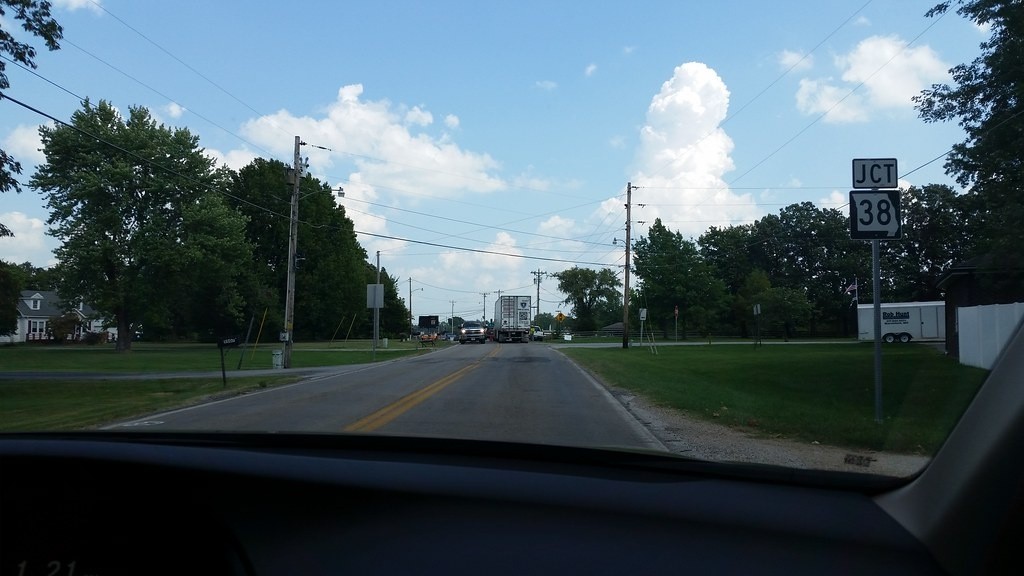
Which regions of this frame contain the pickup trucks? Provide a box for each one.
[530,325,545,342]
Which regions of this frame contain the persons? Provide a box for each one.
[530,325,534,342]
[421,333,435,347]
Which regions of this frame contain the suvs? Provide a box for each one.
[457,320,487,344]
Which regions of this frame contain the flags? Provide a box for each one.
[844,281,856,295]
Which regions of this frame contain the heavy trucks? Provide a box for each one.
[492,295,531,344]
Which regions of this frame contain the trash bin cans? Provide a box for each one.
[383,338,388,349]
[271,350,284,369]
